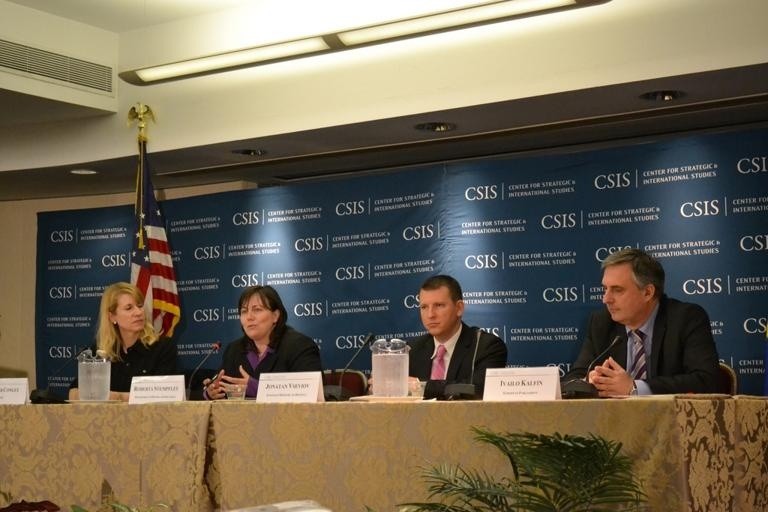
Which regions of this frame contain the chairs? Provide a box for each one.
[322,367,368,402]
[718,362,738,396]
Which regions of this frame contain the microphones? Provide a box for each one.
[188,341,222,391]
[325,332,375,399]
[562,335,622,396]
[444,328,482,397]
[30,347,88,403]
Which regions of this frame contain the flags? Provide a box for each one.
[130,133,181,338]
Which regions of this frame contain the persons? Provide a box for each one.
[368,275,508,400]
[68,282,178,402]
[562,249,719,398]
[202,286,321,401]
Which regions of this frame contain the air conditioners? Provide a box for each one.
[0,39,114,93]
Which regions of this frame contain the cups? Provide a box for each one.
[409,381,427,400]
[226,385,247,401]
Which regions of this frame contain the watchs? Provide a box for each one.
[629,380,638,395]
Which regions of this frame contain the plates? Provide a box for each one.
[64,399,123,404]
[350,395,425,402]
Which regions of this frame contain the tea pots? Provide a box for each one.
[371,339,411,398]
[78,351,110,400]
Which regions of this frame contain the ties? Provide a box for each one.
[628,328,648,380]
[430,344,446,381]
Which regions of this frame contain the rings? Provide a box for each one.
[237,387,241,392]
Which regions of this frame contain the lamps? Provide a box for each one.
[119,36,334,86]
[334,0,612,53]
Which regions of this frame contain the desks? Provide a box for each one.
[0,392,768,512]
